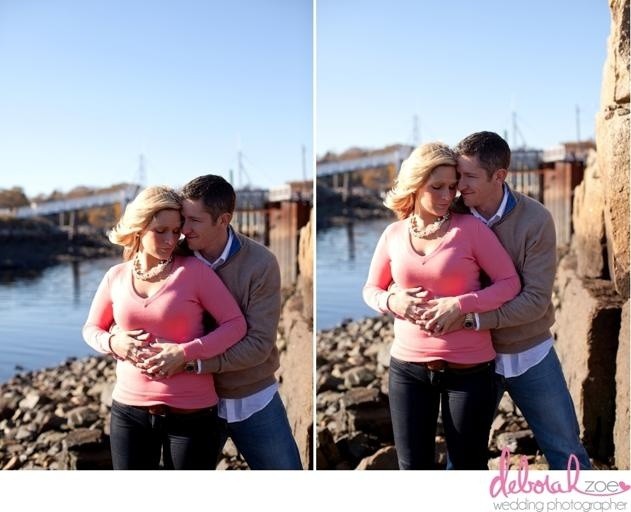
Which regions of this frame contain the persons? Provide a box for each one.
[112,174,303,469]
[82,185,249,469]
[388,131,595,470]
[363,141,524,469]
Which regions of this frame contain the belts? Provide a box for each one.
[416,360,481,372]
[137,405,199,415]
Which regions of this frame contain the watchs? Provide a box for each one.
[184,360,196,373]
[465,313,474,330]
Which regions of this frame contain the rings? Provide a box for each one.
[437,323,443,329]
[159,370,166,376]
[134,349,138,356]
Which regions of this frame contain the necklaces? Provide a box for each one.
[409,210,450,238]
[130,254,173,280]
